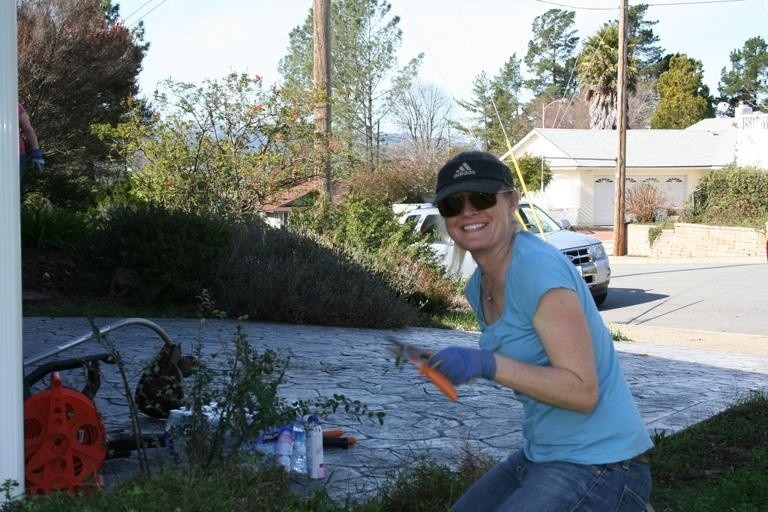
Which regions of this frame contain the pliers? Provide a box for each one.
[383,337,458,401]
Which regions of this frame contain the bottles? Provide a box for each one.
[163,402,265,474]
[273,415,325,478]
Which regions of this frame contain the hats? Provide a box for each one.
[433,158,513,205]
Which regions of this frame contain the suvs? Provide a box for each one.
[397,202,610,306]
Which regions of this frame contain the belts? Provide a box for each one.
[632,454,650,464]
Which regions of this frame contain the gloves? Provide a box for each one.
[429,347,495,386]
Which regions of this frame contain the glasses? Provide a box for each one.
[435,188,517,217]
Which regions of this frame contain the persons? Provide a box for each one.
[427,151,655,512]
[19,105,44,180]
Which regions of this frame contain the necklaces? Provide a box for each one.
[486,246,511,302]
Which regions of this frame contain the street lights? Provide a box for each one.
[542,99,568,129]
